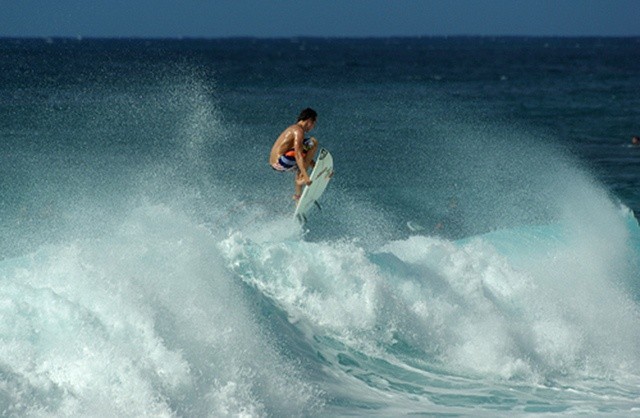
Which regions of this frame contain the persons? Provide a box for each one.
[270,108,333,202]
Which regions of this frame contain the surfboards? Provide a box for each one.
[294,147,333,228]
